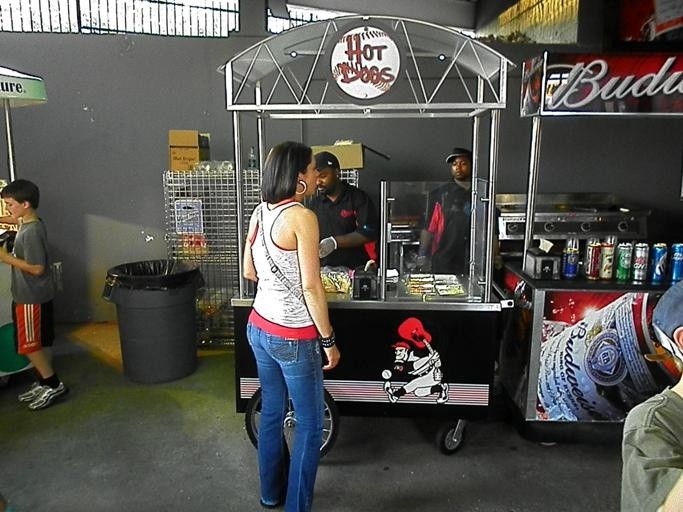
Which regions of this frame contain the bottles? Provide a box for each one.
[248,146,256,169]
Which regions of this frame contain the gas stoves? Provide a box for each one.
[495,192,651,240]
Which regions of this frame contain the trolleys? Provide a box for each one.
[496,51,679,444]
[1,64,49,385]
[215,13,518,458]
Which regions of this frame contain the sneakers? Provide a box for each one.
[17,381,43,402]
[28,381,70,411]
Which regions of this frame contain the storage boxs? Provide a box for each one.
[311,140,392,172]
[167,128,212,172]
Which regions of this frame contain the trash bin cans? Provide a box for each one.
[105,258,199,385]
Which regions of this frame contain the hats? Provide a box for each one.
[313,151,340,171]
[446,147,470,163]
[651,278,683,353]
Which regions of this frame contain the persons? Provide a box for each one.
[384,334,449,404]
[305,152,378,267]
[0,179,69,412]
[418,146,503,276]
[242,141,340,512]
[620,279,683,512]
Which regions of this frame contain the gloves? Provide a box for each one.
[318,235,339,258]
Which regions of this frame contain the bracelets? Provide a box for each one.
[321,331,336,347]
